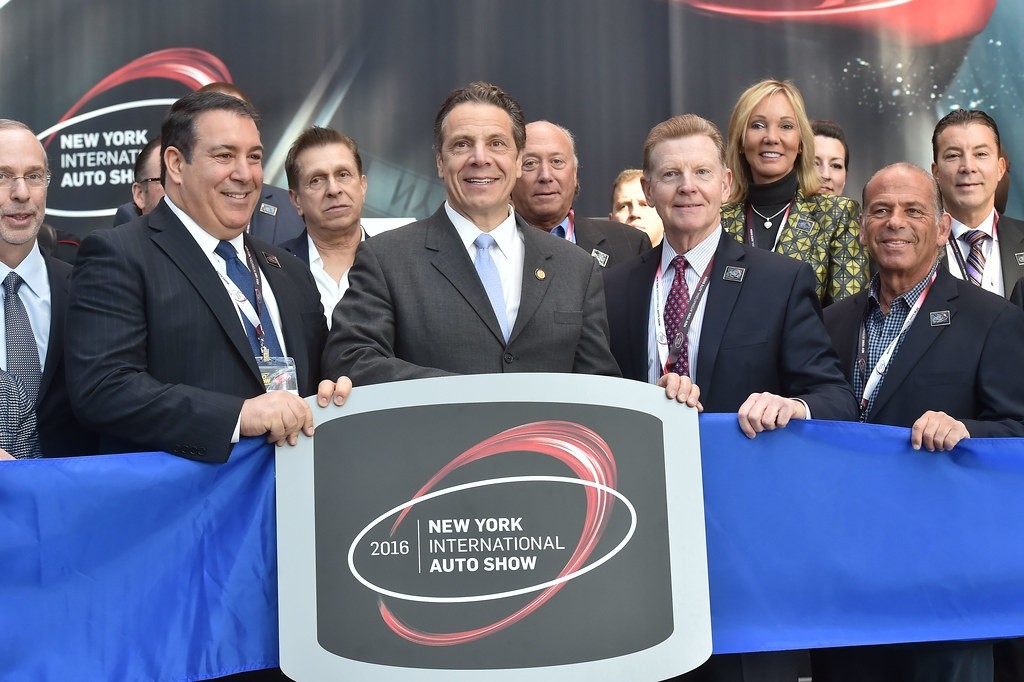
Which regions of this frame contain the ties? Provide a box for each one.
[956,230,991,288]
[214,240,292,392]
[660,255,691,379]
[0,271,42,408]
[472,233,509,345]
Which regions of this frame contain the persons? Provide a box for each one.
[512,120,651,271]
[0,368,42,461]
[609,169,664,248]
[276,127,370,330]
[113,81,308,244]
[721,80,870,310]
[0,119,73,407]
[319,82,623,388]
[131,138,165,215]
[811,119,849,196]
[60,91,350,681]
[931,110,1024,311]
[824,164,1024,682]
[603,114,858,682]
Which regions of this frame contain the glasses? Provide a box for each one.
[0,171,51,188]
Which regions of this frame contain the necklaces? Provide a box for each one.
[750,202,791,229]
